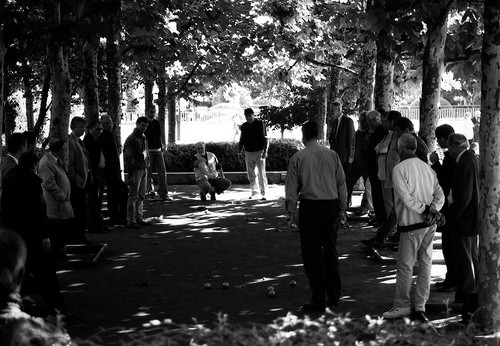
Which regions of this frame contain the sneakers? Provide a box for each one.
[249,194,258,199]
[262,196,266,200]
[415,304,426,312]
[383,306,411,318]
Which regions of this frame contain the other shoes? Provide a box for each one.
[201,193,206,201]
[211,194,216,200]
[435,275,462,291]
[345,206,399,251]
[87,189,175,232]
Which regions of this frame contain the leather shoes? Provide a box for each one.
[302,301,338,314]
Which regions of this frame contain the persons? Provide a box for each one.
[62,116,94,246]
[0,132,29,200]
[284,121,348,312]
[435,132,482,314]
[82,118,114,235]
[94,114,132,228]
[188,141,232,202]
[122,117,154,230]
[325,99,356,182]
[344,110,429,249]
[0,228,70,345]
[428,124,465,293]
[35,135,76,274]
[0,152,50,295]
[236,107,271,201]
[382,132,446,320]
[21,130,34,152]
[142,105,174,203]
